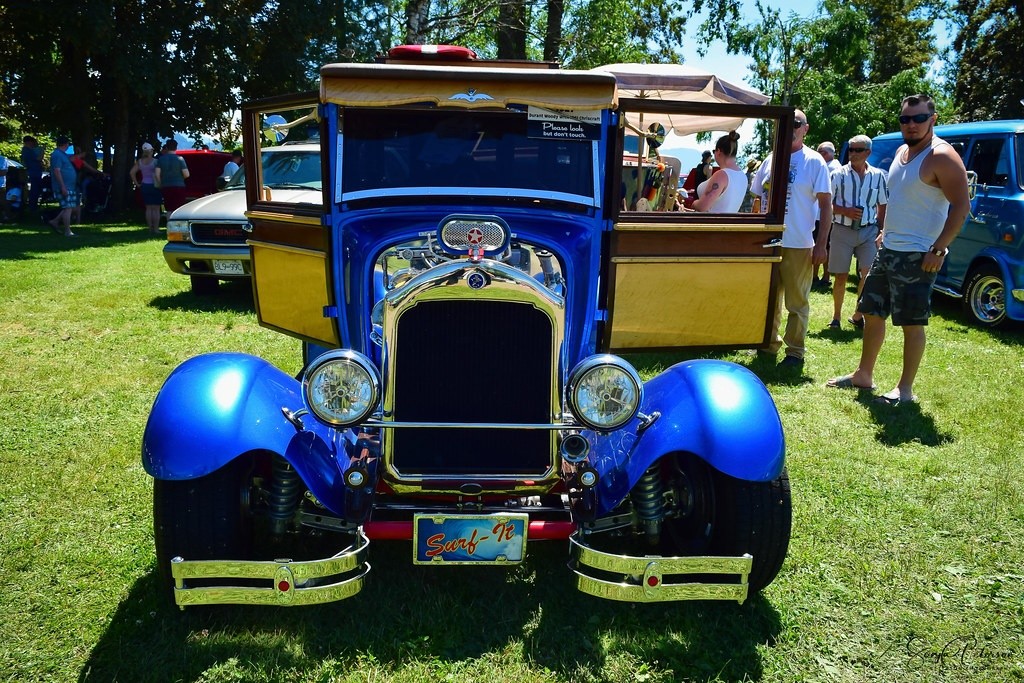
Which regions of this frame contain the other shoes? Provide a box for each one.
[776,356,804,368]
[64,233,79,237]
[848,316,865,328]
[49,221,63,234]
[752,352,775,367]
[827,319,839,328]
[155,229,164,235]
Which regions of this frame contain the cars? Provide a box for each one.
[163,139,410,298]
[141,44,791,612]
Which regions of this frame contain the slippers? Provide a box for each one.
[826,376,876,389]
[870,395,918,406]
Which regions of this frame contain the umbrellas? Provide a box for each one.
[590,62,772,205]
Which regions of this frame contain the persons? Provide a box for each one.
[690,129,748,213]
[673,186,688,210]
[811,140,842,287]
[750,108,833,370]
[154,138,189,223]
[694,148,712,198]
[222,149,242,180]
[828,132,887,330]
[128,142,162,234]
[826,95,970,407]
[0,134,108,237]
[740,157,761,212]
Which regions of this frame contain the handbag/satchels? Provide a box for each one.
[151,159,160,188]
[752,198,760,212]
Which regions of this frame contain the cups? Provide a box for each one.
[851,205,865,231]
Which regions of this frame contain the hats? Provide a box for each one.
[57,136,70,142]
[233,150,242,156]
[702,150,713,156]
[142,142,155,150]
[23,136,34,141]
[746,158,762,172]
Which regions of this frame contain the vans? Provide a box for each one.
[840,120,1024,331]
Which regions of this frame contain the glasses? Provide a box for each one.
[898,112,934,124]
[713,149,716,153]
[847,146,868,153]
[793,121,806,129]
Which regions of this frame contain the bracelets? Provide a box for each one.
[929,244,948,256]
